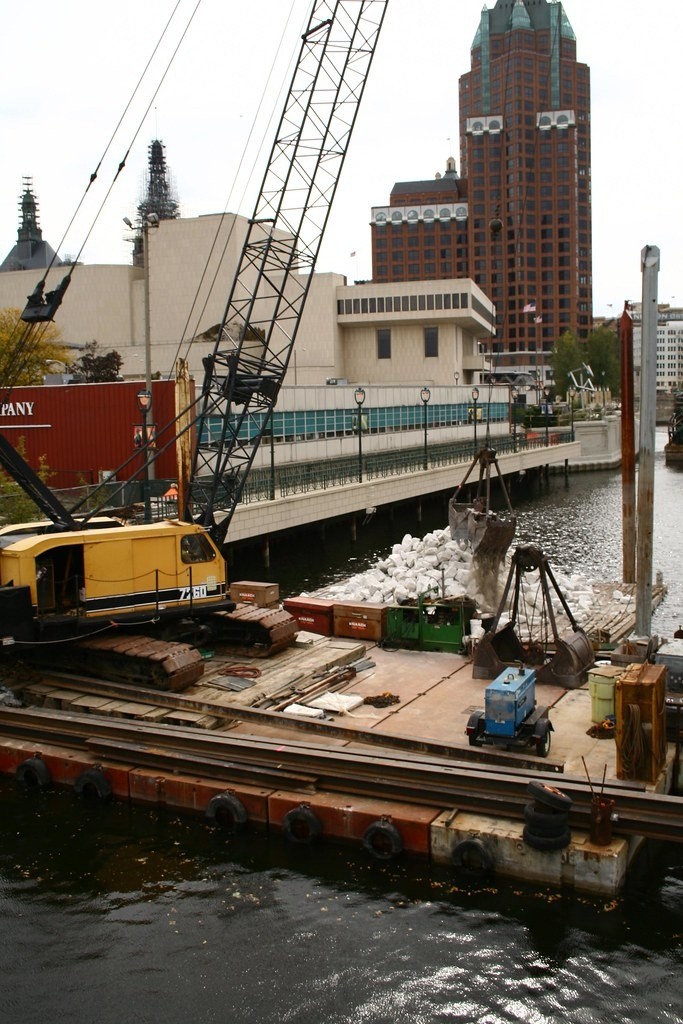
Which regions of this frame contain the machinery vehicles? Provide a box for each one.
[568,388,576,442]
[0,1,374,698]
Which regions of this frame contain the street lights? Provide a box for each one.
[511,387,519,455]
[544,386,551,447]
[355,388,366,483]
[471,386,479,462]
[419,387,431,471]
